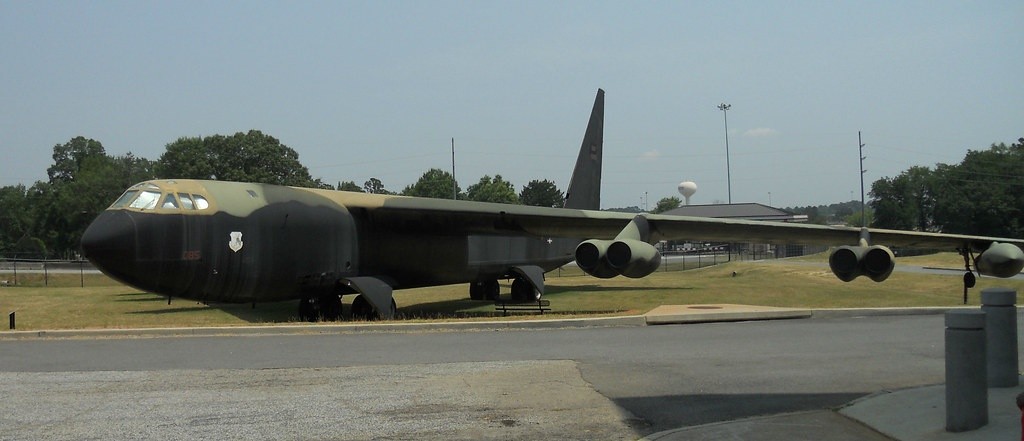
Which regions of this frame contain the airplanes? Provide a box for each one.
[80,89,1024,322]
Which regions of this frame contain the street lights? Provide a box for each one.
[716,103,732,203]
